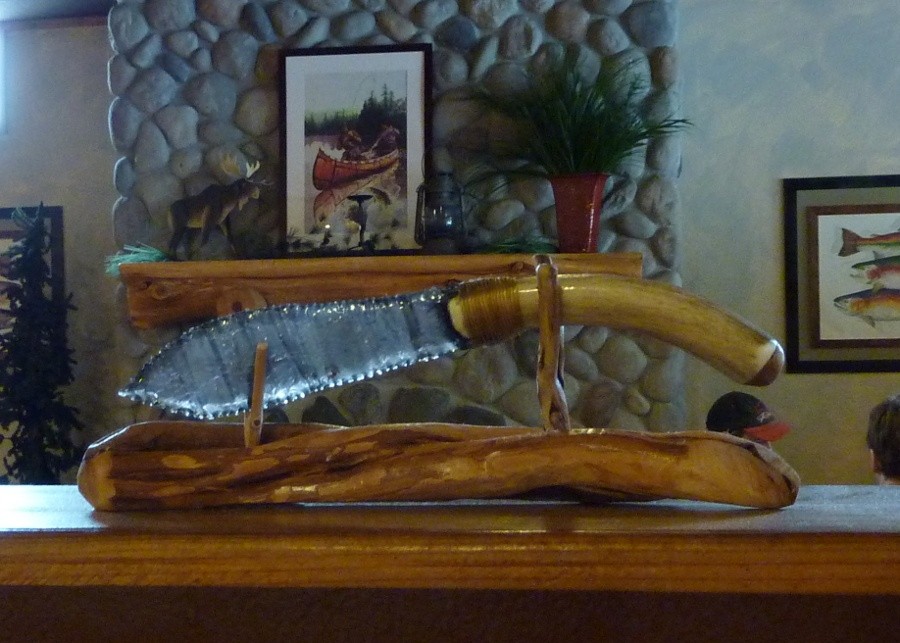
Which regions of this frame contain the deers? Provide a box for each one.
[165,151,278,262]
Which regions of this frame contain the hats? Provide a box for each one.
[706,391,790,440]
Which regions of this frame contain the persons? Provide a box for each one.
[866,395,900,486]
[704,390,791,451]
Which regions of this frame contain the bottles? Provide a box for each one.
[415,171,466,255]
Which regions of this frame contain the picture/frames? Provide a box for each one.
[3,205,66,367]
[782,174,900,373]
[278,38,431,257]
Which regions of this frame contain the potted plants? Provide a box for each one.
[464,49,698,252]
